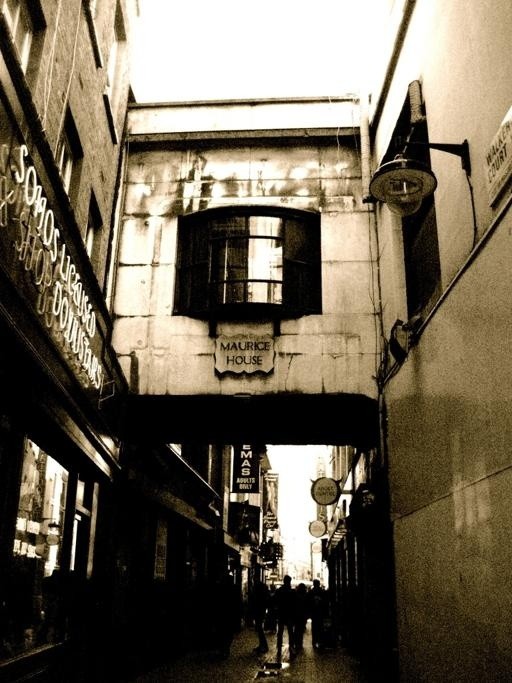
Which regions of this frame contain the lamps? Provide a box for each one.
[367,134,472,216]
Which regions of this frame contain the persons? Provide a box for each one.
[247,573,328,660]
